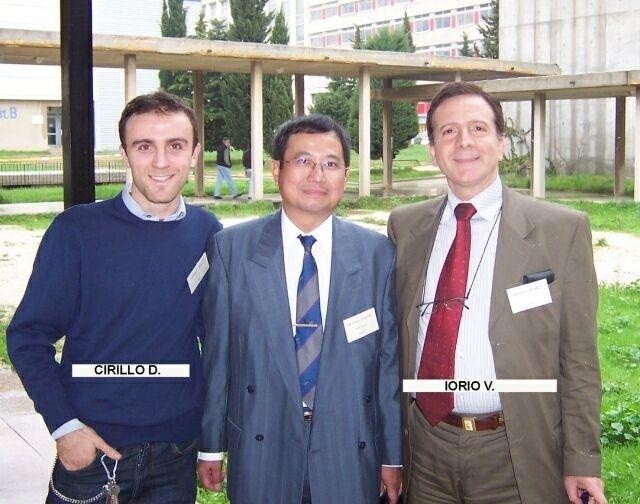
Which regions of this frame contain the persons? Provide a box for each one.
[385,79,608,504]
[213,137,243,200]
[241,141,264,199]
[194,112,408,504]
[6,92,223,504]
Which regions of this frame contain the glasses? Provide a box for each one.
[415,295,470,318]
[281,157,348,171]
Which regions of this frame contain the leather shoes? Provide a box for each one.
[213,196,224,199]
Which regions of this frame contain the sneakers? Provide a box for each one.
[233,192,245,199]
[438,411,505,431]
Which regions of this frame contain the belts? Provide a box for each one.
[303,412,314,422]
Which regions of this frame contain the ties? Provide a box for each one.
[414,203,479,428]
[294,235,325,411]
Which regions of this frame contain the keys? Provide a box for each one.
[104,482,121,504]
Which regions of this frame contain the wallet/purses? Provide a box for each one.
[523,270,556,285]
